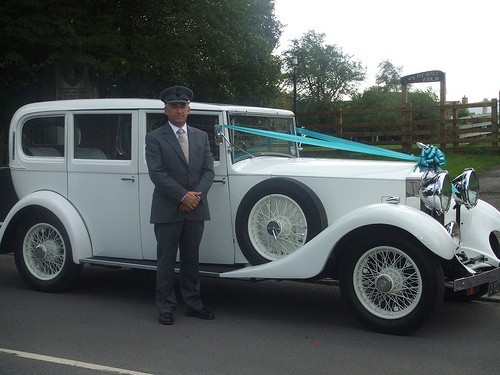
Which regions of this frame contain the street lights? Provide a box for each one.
[291,56,298,127]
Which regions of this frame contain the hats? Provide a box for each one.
[160,85,194,104]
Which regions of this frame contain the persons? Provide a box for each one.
[145,86,216,324]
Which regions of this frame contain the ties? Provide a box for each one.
[177,129,190,164]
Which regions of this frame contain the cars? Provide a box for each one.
[0,96,500,336]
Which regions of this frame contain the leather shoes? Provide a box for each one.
[158,312,174,325]
[185,308,215,320]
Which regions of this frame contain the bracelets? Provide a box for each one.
[197,195,201,199]
